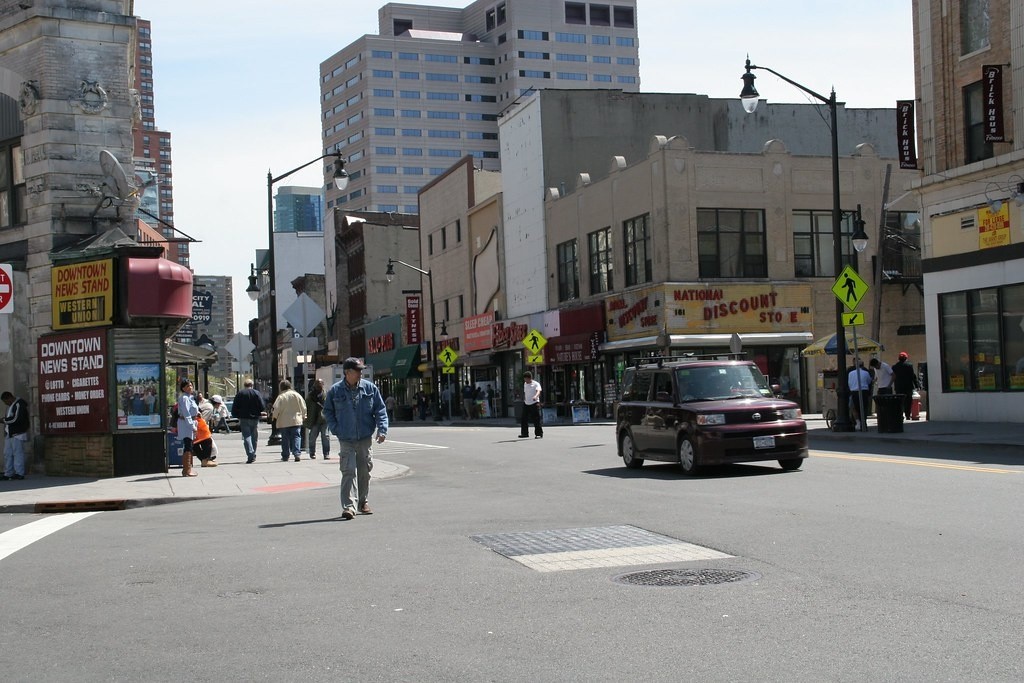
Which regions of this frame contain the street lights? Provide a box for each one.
[385,257,449,418]
[246,148,349,445]
[740,54,869,429]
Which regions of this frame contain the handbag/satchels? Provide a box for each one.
[193,416,212,444]
[170,410,178,427]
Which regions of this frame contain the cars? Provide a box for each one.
[213,401,241,432]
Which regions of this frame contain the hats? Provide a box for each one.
[898,351,909,358]
[343,357,368,369]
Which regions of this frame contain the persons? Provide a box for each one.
[120,380,157,415]
[233,380,266,464]
[892,352,917,420]
[847,357,872,430]
[870,358,895,393]
[175,380,231,478]
[413,381,494,420]
[305,379,331,460]
[518,371,543,439]
[273,380,308,462]
[323,357,388,518]
[384,394,397,423]
[0,392,30,481]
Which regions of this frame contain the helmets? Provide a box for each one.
[210,395,222,404]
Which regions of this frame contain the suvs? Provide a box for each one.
[615,352,808,477]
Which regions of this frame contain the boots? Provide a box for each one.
[182,451,198,476]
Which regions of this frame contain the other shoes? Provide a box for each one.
[324,456,330,460]
[0,474,25,480]
[535,435,541,439]
[295,456,300,461]
[246,454,257,463]
[342,508,356,520]
[209,456,216,460]
[518,434,529,437]
[201,458,217,467]
[310,452,316,459]
[281,456,288,461]
[358,503,373,514]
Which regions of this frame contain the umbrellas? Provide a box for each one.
[801,331,885,358]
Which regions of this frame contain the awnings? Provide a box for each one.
[391,344,419,379]
